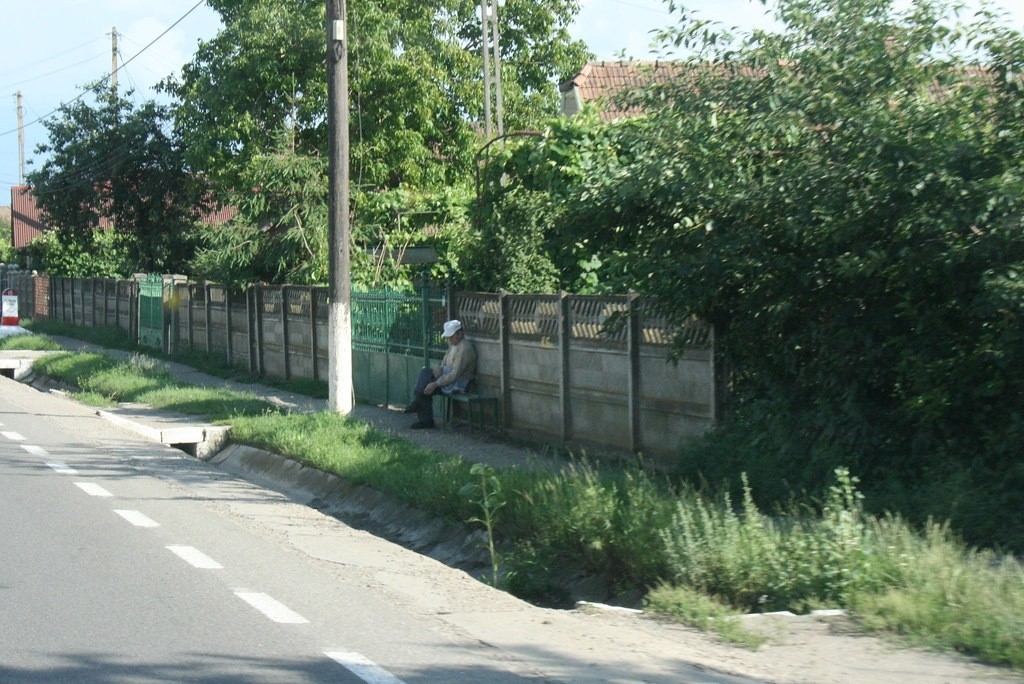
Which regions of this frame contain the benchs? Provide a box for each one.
[434,393,500,437]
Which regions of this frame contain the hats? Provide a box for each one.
[442,320,461,336]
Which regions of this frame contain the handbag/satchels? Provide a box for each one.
[441,366,468,392]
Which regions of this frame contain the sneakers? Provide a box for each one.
[410,419,435,429]
[404,401,421,413]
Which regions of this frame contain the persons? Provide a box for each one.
[404,320,476,429]
